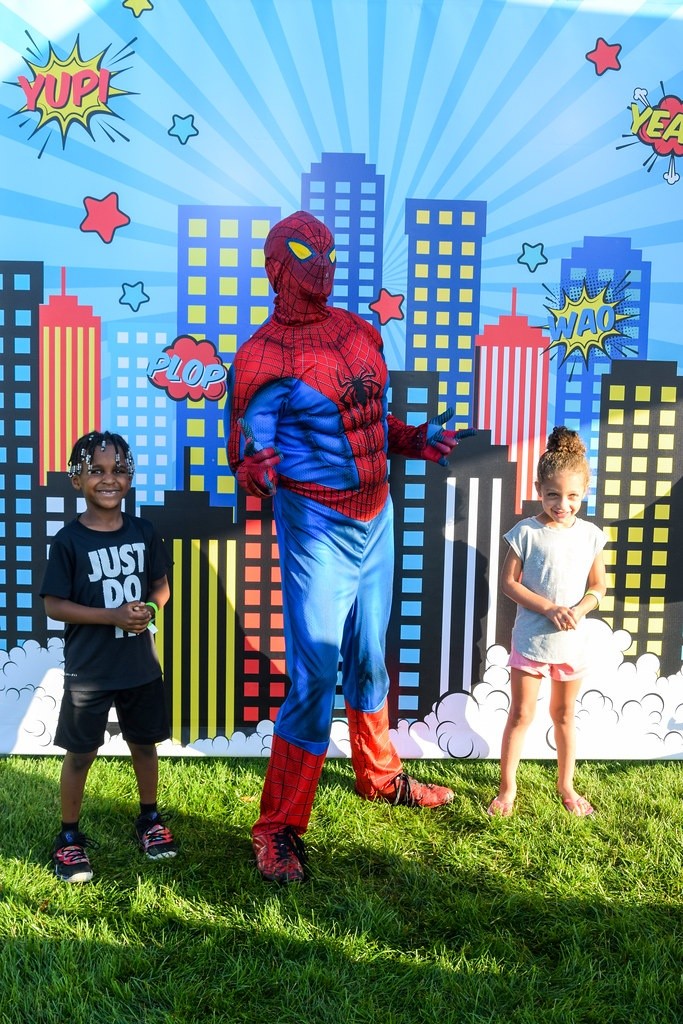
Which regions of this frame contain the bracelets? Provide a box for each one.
[585,590,601,610]
[146,602,158,615]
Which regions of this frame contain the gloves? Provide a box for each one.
[237,417,284,491]
[420,407,478,466]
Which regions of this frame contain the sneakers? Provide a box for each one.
[252,826,308,882]
[372,772,454,808]
[135,811,178,860]
[49,830,100,884]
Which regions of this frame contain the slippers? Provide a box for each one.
[563,796,593,815]
[487,796,514,818]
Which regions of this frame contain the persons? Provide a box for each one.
[38,430,175,884]
[227,210,478,884]
[487,426,610,818]
